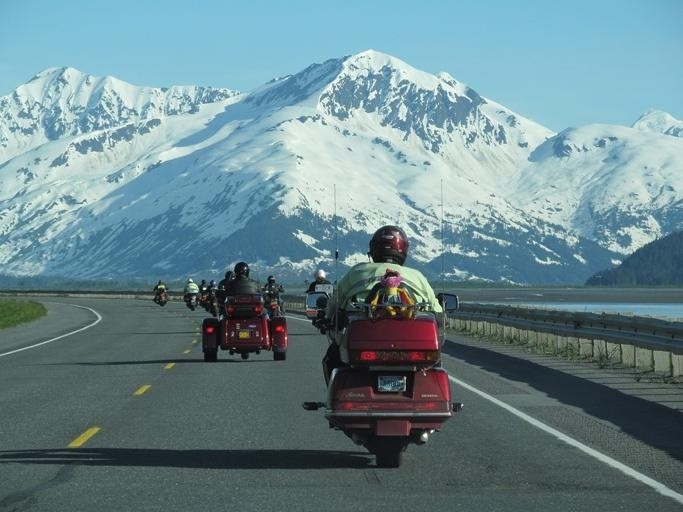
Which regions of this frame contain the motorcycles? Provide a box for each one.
[152,286,170,307]
[181,291,201,310]
[302,288,465,466]
[199,290,288,364]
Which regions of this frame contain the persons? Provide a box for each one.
[324,225,447,381]
[304,269,332,299]
[183,262,286,321]
[153,280,168,300]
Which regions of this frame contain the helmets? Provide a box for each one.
[268,276,275,282]
[234,262,250,277]
[158,280,162,283]
[202,280,206,284]
[369,224,409,265]
[225,271,235,280]
[316,269,325,279]
[188,278,193,283]
[210,280,216,287]
